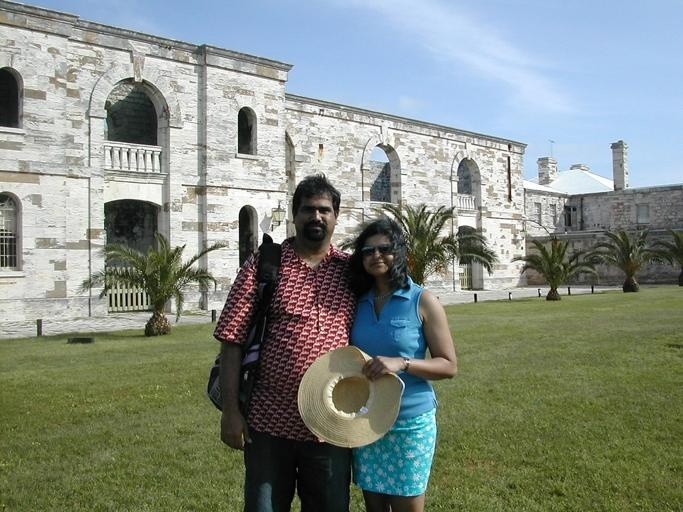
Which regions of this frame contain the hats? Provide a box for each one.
[298,345,405,447]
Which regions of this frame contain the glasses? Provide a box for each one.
[360,244,394,256]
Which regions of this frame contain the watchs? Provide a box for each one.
[403,357,411,373]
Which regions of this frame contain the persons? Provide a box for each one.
[349,218,457,512]
[212,175,358,512]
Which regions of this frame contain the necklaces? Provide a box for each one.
[371,287,399,300]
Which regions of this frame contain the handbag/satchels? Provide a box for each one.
[207,233,282,419]
